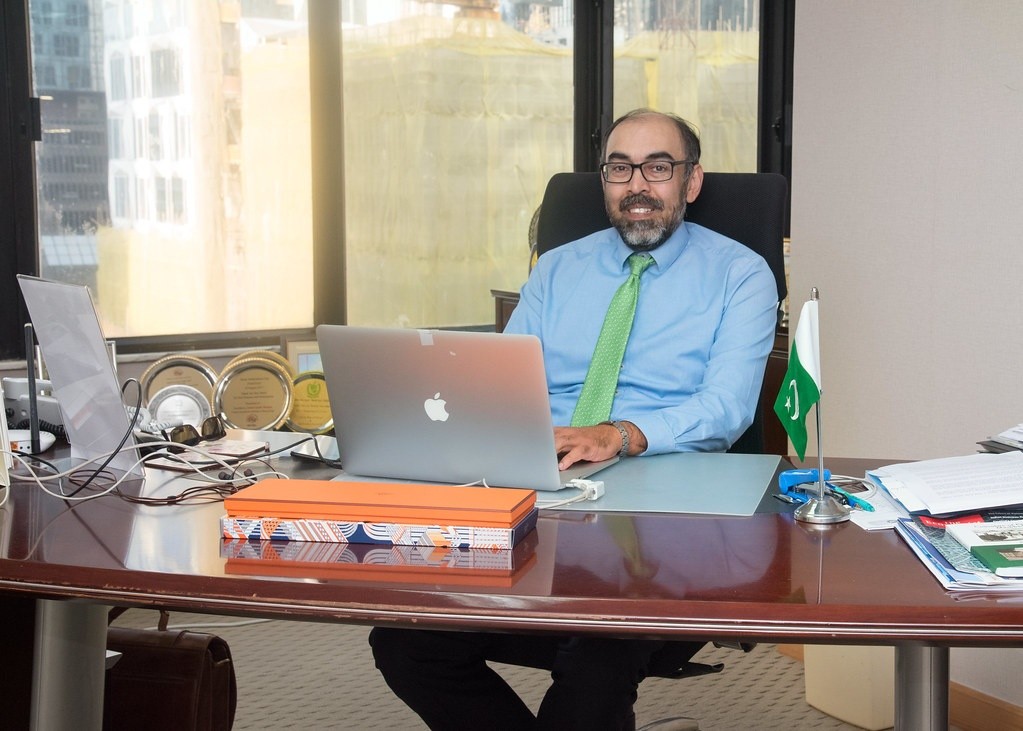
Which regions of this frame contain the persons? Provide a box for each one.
[369,110,781,731]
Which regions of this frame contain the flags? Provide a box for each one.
[775,299,824,466]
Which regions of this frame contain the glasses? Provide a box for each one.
[600,160,692,184]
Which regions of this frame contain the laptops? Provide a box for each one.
[316,324,620,491]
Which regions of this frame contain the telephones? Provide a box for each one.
[0,377,63,436]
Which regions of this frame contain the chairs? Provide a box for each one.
[481,170,795,731]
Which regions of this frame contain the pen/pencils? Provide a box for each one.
[826,481,864,511]
[825,488,849,506]
[828,481,876,512]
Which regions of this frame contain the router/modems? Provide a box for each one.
[7,324,55,456]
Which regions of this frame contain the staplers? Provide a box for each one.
[777,468,832,504]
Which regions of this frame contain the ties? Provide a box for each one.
[570,252,655,428]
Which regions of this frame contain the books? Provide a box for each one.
[844,420,1023,591]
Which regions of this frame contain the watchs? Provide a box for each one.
[597,421,630,457]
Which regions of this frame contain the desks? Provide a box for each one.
[0,429,1022,730]
